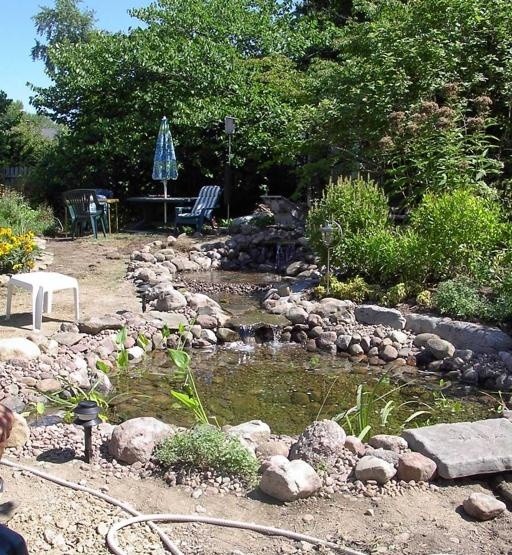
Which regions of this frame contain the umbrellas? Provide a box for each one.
[152,116,180,224]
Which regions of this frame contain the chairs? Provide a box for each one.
[61,183,225,240]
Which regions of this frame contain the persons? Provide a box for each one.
[1,390,29,555]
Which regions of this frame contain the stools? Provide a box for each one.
[5,271,81,332]
